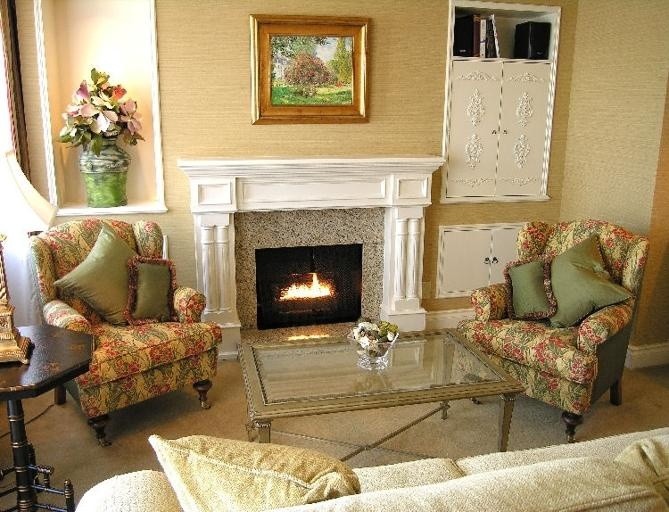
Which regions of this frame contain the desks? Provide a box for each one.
[1,324,97,511]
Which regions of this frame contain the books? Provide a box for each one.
[479,19,486,57]
[487,21,496,58]
[481,14,500,58]
[452,15,479,57]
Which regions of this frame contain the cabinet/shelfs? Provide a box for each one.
[439,0,562,206]
[434,222,528,298]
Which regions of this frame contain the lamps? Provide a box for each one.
[0,150,62,367]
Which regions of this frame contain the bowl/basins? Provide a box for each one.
[354,334,399,356]
[355,355,393,371]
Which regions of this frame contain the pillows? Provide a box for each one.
[50,222,142,326]
[123,254,180,326]
[502,251,558,323]
[145,430,362,512]
[548,232,637,330]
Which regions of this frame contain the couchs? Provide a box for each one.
[28,219,224,448]
[73,422,669,512]
[458,217,653,443]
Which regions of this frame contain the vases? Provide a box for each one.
[77,137,132,209]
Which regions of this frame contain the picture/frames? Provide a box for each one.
[247,12,373,127]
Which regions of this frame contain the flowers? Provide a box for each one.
[57,67,147,155]
